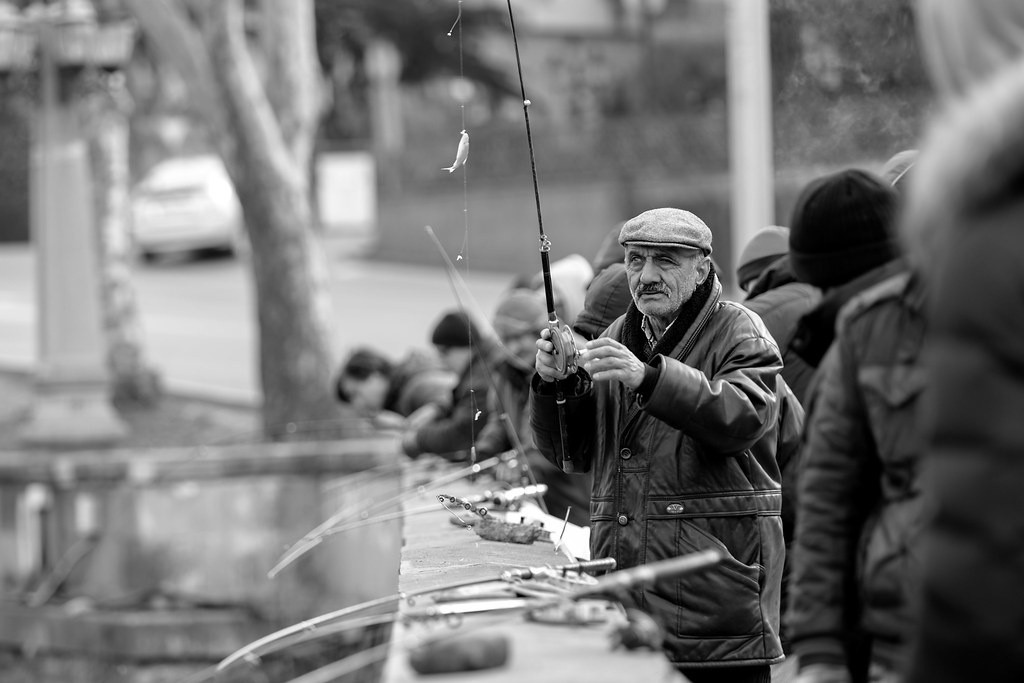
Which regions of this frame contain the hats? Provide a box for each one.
[618,208,713,256]
[789,170,899,287]
[432,311,480,346]
[882,150,922,188]
[737,225,790,292]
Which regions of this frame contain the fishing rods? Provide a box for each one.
[506,1,578,476]
[263,222,552,582]
[216,545,725,683]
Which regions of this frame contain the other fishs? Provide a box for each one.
[441,130,470,175]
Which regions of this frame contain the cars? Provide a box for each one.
[129,153,242,266]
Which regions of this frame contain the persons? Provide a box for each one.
[335,0,1024,682]
